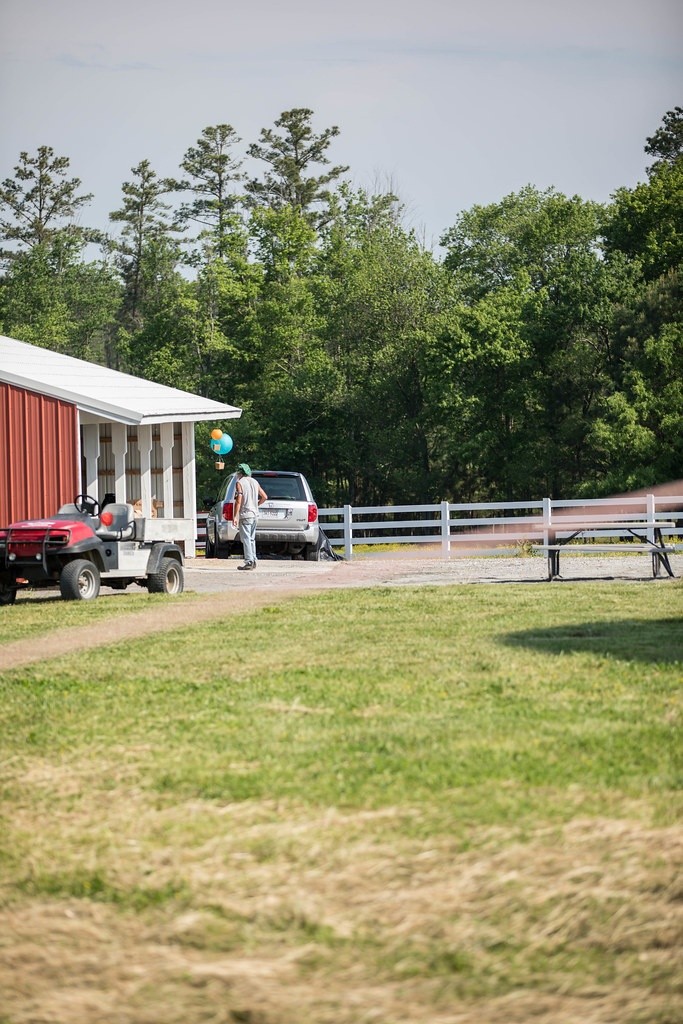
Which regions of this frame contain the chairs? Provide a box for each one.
[94,503,134,541]
[59,503,92,515]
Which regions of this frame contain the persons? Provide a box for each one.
[232,463,268,571]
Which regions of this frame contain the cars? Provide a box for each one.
[204,470,320,560]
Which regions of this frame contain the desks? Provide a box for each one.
[531,522,675,581]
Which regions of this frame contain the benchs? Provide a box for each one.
[531,544,673,578]
[554,543,675,579]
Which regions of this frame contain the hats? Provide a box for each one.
[236,463,251,476]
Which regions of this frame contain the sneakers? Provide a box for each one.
[237,561,257,570]
